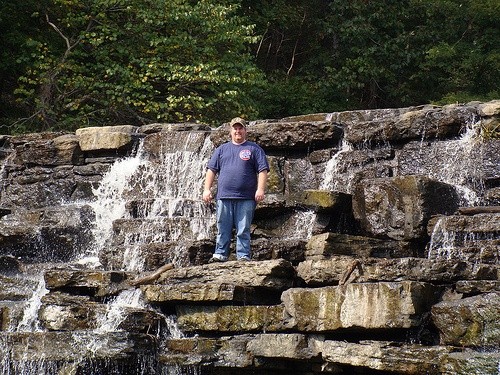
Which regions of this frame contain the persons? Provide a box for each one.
[202,117,270,262]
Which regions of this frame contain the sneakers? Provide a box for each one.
[239,258,250,261]
[212,253,229,262]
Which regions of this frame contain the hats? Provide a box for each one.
[231,117,246,127]
